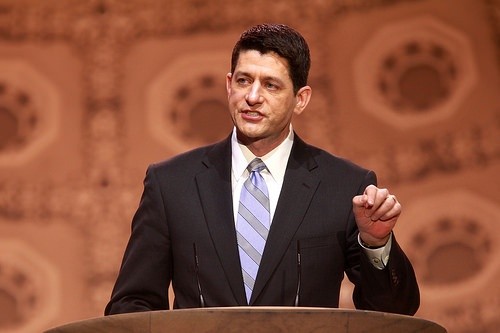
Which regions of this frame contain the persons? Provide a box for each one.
[104,23,421,317]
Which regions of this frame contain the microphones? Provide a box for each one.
[294,239,301,307]
[193,241,205,308]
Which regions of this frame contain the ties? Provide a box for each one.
[236,158,270,304]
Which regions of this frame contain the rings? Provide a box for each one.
[389,196,396,201]
